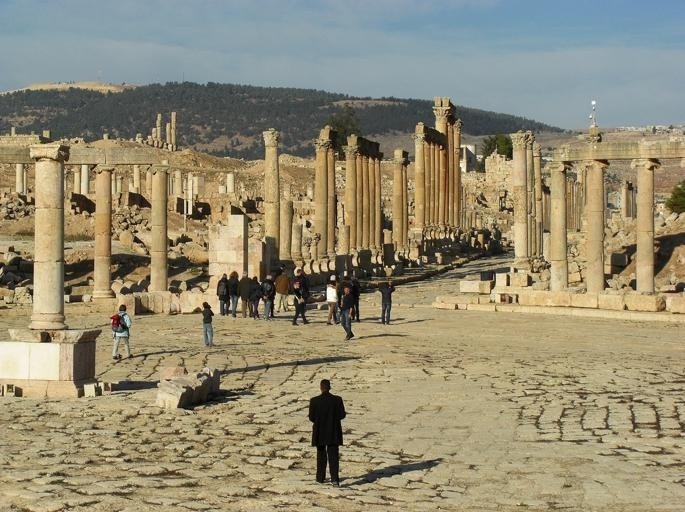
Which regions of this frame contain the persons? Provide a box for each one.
[259,275,275,320]
[309,379,345,485]
[292,280,310,326]
[295,268,310,311]
[201,302,214,346]
[216,274,230,316]
[111,304,133,358]
[270,300,275,319]
[228,271,240,317]
[237,271,253,317]
[250,276,263,319]
[275,271,291,312]
[325,275,361,340]
[380,283,395,325]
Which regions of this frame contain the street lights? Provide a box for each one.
[589,99,600,126]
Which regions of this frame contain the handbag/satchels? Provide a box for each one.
[297,298,305,303]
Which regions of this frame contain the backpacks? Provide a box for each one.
[111,313,126,333]
